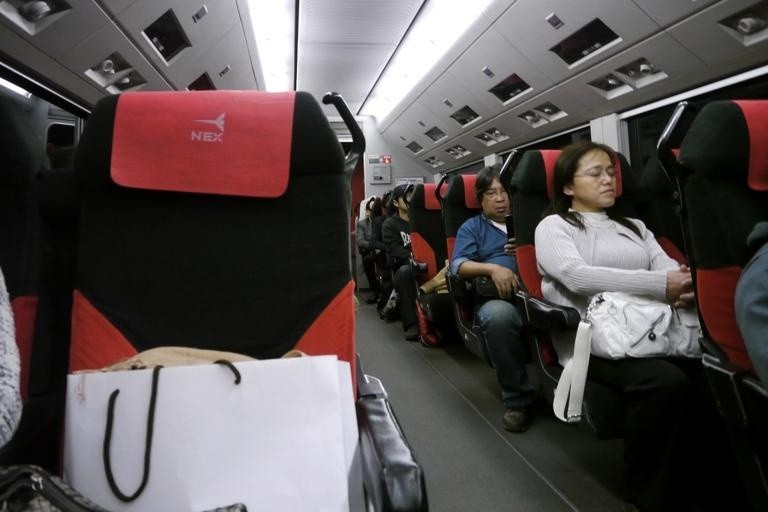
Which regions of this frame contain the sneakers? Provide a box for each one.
[404,322,420,341]
[501,403,535,433]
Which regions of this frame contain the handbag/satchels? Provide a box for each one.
[585,290,705,360]
[0,463,247,512]
[60,350,367,512]
[473,269,520,299]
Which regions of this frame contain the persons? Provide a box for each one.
[349,189,401,321]
[533,140,749,512]
[380,184,422,342]
[446,163,537,435]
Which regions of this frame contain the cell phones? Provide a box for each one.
[504,215,515,241]
